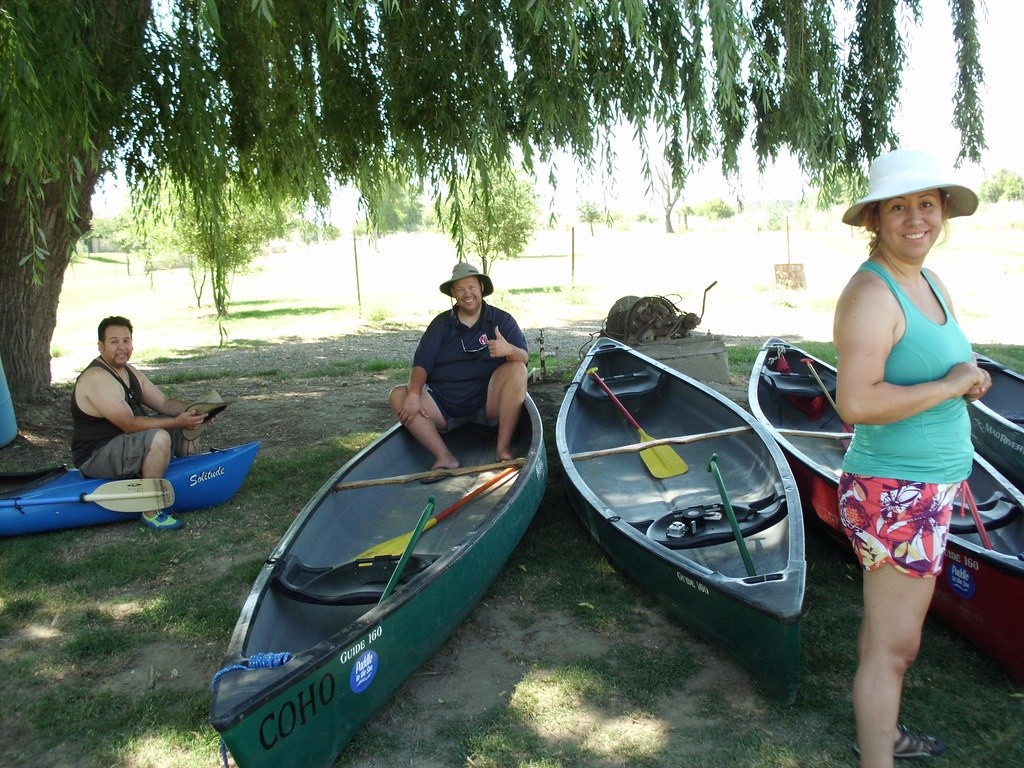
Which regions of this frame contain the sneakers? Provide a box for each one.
[141,510,184,531]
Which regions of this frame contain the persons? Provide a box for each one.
[833,149,991,768]
[387,263,529,484]
[71,316,208,530]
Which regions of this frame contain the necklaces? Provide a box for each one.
[99,355,133,394]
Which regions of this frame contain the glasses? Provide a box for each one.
[460,333,489,353]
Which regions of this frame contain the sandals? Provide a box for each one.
[853,723,947,760]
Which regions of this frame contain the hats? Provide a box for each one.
[180,389,228,442]
[439,263,494,299]
[842,146,979,227]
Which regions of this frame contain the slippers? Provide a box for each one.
[495,457,519,470]
[421,467,451,483]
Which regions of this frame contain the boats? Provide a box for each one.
[0,441,266,538]
[746,336,1024,686]
[553,336,808,707]
[209,389,551,768]
[965,349,1022,494]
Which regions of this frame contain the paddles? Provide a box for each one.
[960,474,995,553]
[348,460,522,567]
[0,475,178,513]
[585,364,691,480]
[798,356,856,450]
[376,493,448,604]
[707,451,758,577]
